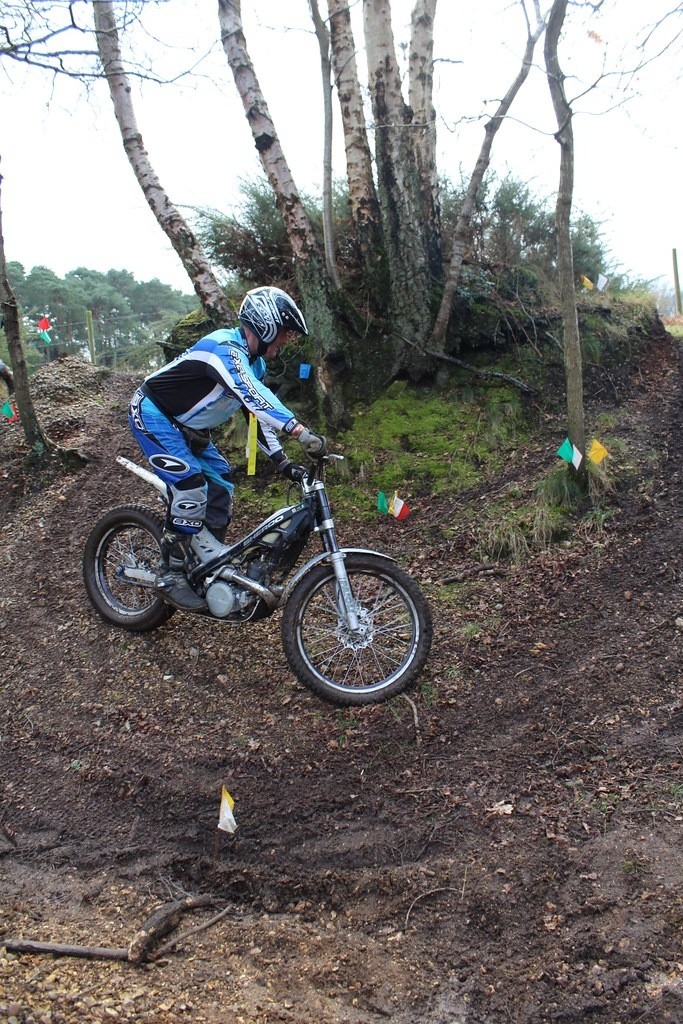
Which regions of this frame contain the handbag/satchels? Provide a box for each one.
[140,381,211,455]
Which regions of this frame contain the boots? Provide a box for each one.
[153,526,206,611]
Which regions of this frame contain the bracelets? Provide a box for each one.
[294,424,303,439]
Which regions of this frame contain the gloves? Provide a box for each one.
[278,460,309,484]
[296,428,328,463]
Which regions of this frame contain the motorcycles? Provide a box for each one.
[79,435,435,708]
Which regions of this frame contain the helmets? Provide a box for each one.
[238,285,309,345]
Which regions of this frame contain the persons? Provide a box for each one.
[125,285,321,614]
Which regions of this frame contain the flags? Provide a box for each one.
[39,330,52,344]
[556,437,574,464]
[583,276,593,290]
[596,274,607,291]
[37,318,51,331]
[6,405,18,422]
[0,401,15,418]
[571,443,583,470]
[377,491,390,515]
[388,491,409,520]
[587,438,608,465]
